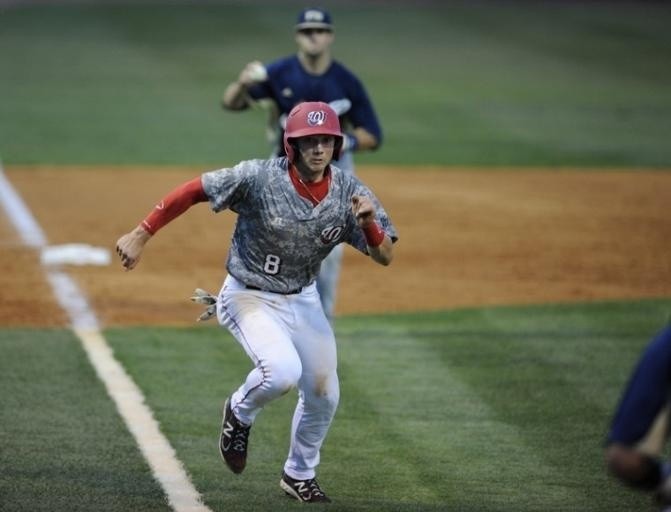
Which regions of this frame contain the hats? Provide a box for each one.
[296,8,332,31]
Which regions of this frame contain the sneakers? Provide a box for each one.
[219,396,251,474]
[279,471,330,503]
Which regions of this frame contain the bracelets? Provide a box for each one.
[632,458,660,490]
[348,133,358,151]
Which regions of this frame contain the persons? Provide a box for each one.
[221,9,384,325]
[600,317,671,512]
[114,102,400,504]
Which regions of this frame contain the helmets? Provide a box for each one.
[283,101,343,163]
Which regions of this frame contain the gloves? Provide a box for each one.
[189,288,217,322]
